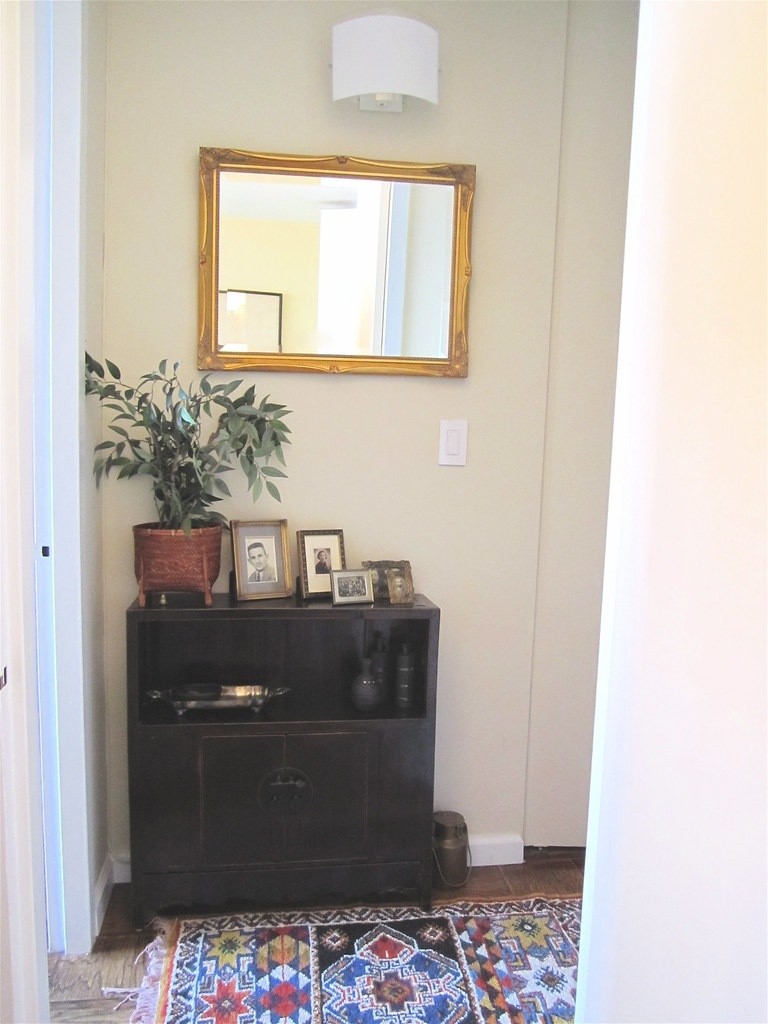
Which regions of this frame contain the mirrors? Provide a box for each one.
[196,145,478,382]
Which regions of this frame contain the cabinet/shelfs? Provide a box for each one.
[124,590,441,930]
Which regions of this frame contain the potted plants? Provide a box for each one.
[82,352,292,608]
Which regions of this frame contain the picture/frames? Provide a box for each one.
[230,517,291,601]
[330,569,374,607]
[295,528,346,599]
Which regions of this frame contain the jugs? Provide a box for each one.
[433,810,472,889]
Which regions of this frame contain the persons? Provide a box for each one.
[247,542,276,582]
[316,551,329,574]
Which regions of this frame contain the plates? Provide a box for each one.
[147,680,289,715]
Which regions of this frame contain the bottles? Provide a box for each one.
[344,635,417,708]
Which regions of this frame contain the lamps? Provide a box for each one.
[332,13,439,113]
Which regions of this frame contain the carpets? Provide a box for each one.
[101,892,583,1024]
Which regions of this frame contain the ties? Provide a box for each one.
[257,571,260,582]
